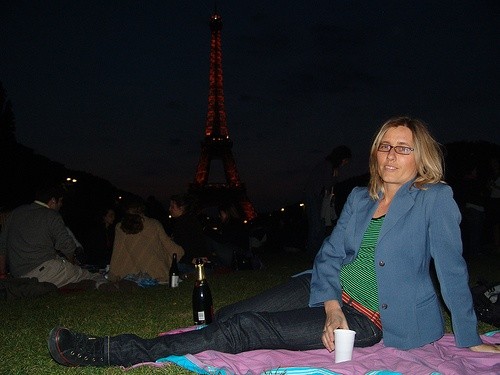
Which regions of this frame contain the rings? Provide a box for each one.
[322,330,327,333]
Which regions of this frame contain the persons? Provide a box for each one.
[1,171,210,288]
[47,116,500,367]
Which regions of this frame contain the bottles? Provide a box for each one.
[191,258,215,325]
[169,253,180,288]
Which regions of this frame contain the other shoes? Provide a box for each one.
[95,281,114,290]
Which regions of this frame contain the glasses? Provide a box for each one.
[378,143,414,154]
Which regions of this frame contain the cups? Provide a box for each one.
[333,329,356,363]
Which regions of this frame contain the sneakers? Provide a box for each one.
[48,327,104,367]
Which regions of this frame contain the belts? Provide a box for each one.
[342,290,382,330]
